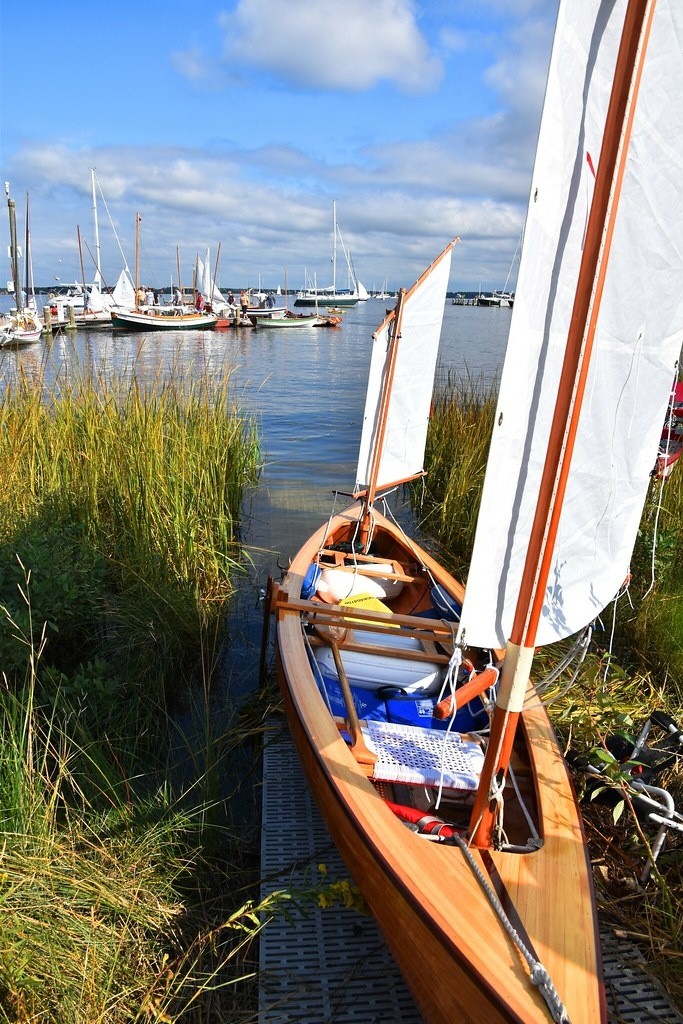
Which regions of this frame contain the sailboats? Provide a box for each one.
[0,169,526,349]
[259,1,682,1024]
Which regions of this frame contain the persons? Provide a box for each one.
[227,291,236,305]
[172,288,182,316]
[240,290,251,319]
[81,287,97,320]
[264,292,276,308]
[138,285,165,316]
[13,287,27,307]
[195,290,203,312]
